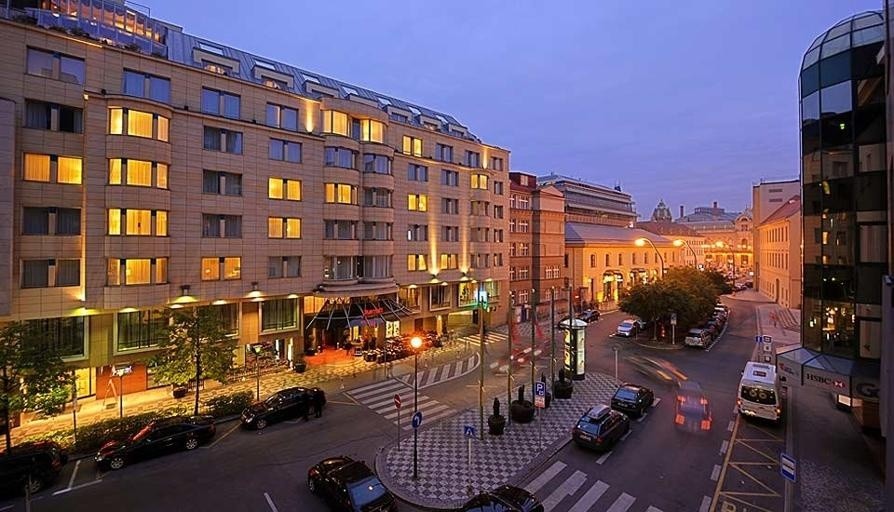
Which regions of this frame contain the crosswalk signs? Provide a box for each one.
[464,424,476,439]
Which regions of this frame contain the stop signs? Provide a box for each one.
[393,393,402,409]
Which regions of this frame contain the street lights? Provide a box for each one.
[635,238,664,283]
[411,335,422,479]
[673,239,698,271]
[715,241,735,287]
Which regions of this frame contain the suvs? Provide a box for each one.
[0,440,68,498]
[462,485,545,512]
[307,455,400,512]
[571,403,629,455]
[610,383,655,418]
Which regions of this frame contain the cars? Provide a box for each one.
[94,414,217,471]
[617,318,641,338]
[239,386,327,430]
[731,283,747,291]
[684,303,728,349]
[578,310,600,323]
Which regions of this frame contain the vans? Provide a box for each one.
[672,380,712,435]
[737,361,787,423]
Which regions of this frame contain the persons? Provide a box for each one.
[345,336,376,356]
[312,389,326,418]
[302,389,311,421]
